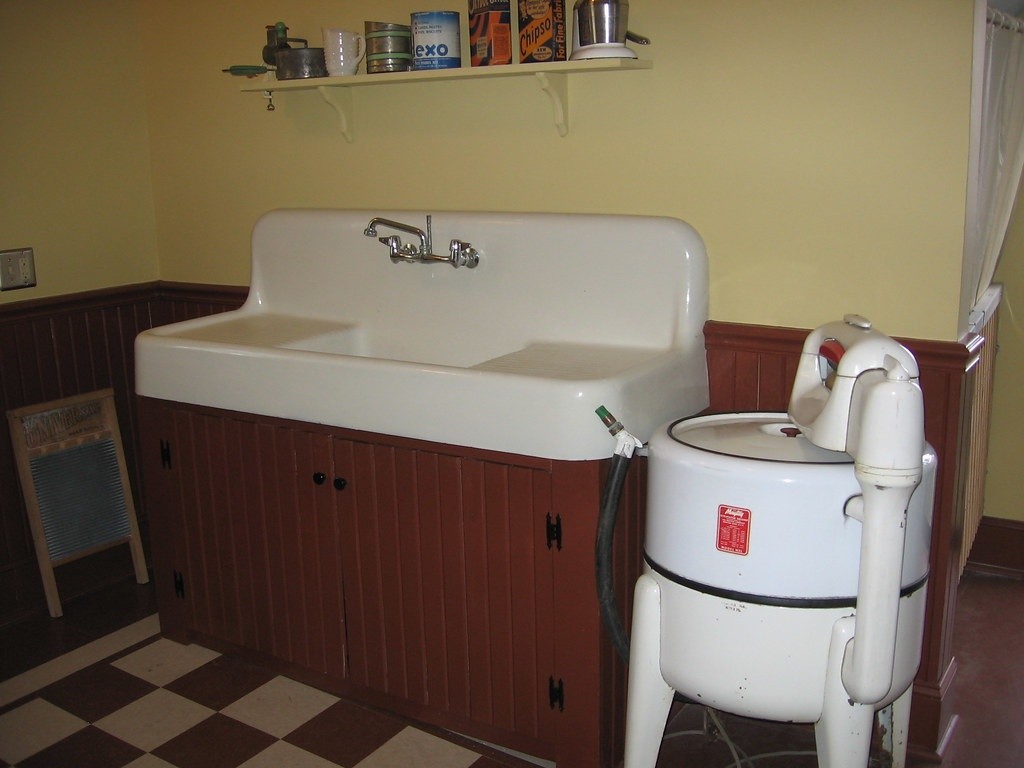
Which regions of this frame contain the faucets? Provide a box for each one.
[364,217,480,269]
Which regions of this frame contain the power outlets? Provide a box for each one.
[1,247,37,291]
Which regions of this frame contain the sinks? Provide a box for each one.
[279,323,534,368]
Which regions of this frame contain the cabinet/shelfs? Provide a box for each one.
[134,396,646,768]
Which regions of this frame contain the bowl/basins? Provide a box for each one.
[275,47,328,80]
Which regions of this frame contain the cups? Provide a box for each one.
[322,27,366,77]
[364,20,412,73]
